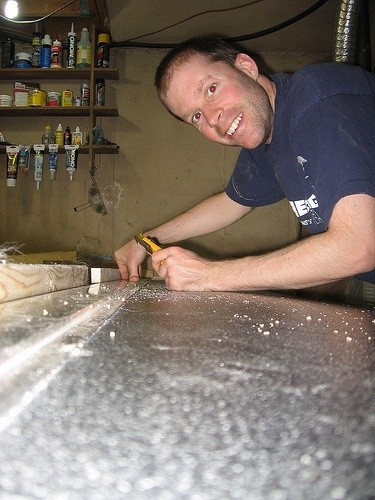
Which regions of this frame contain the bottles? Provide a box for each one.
[56,124,64,145]
[72,125,82,144]
[42,123,55,143]
[51,40,63,68]
[81,83,90,106]
[77,28,91,68]
[32,32,41,68]
[94,79,106,106]
[66,23,77,68]
[64,127,72,145]
[2,37,15,68]
[41,35,51,69]
[96,16,111,67]
[62,91,72,106]
[93,126,103,144]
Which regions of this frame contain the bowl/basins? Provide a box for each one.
[0,95,12,107]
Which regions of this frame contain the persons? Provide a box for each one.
[114,36,375,292]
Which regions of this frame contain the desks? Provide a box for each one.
[0,260,375,500]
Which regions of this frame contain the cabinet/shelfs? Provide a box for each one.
[0,0,119,174]
